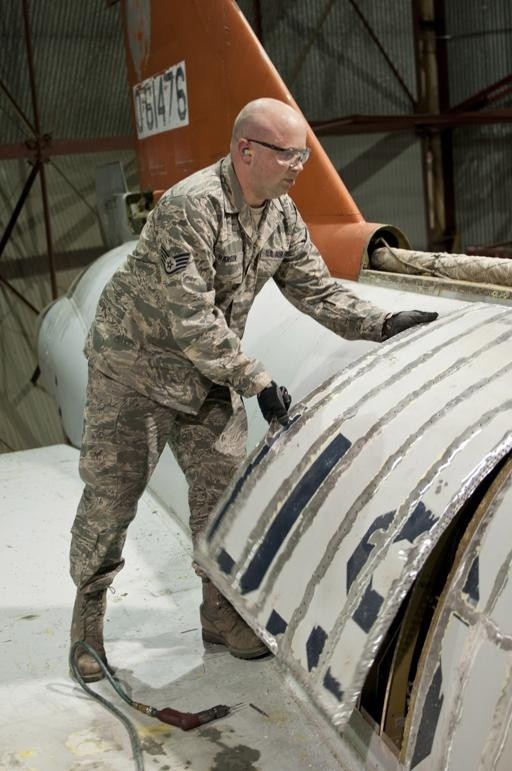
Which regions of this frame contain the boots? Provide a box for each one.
[70,590,106,683]
[200,581,270,661]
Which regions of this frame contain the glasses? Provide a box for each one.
[247,138,311,164]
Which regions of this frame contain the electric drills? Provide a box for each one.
[157,699,247,732]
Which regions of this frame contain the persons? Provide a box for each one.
[69,97,440,682]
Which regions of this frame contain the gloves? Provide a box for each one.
[256,381,292,426]
[383,310,437,337]
